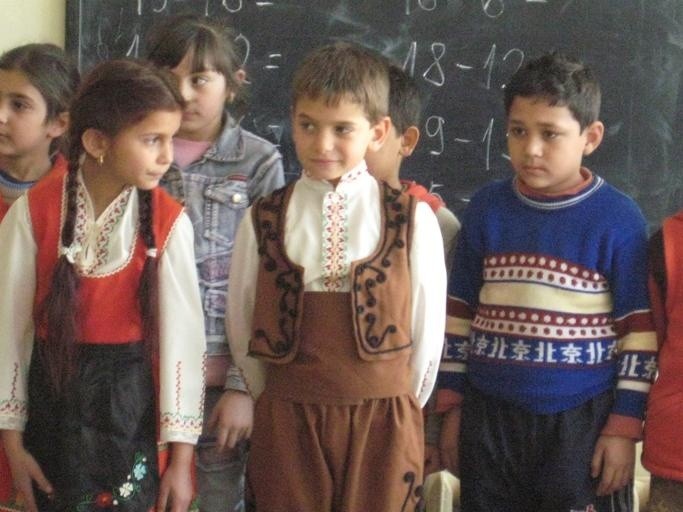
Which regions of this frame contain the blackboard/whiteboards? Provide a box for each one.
[66,0,683,241]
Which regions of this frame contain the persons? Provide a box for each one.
[366,58,464,509]
[0,43,78,218]
[637,207,682,512]
[434,51,658,511]
[227,42,450,511]
[138,14,285,509]
[0,60,209,511]
